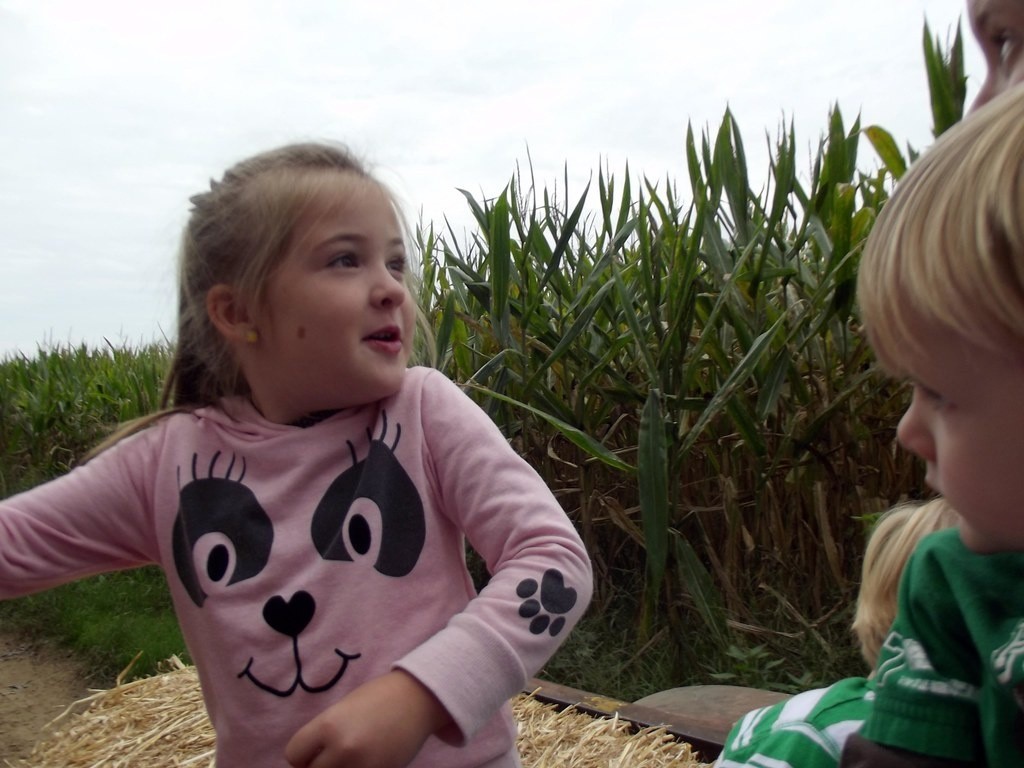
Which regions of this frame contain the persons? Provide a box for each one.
[0,142,593,768]
[708,0,1024,768]
